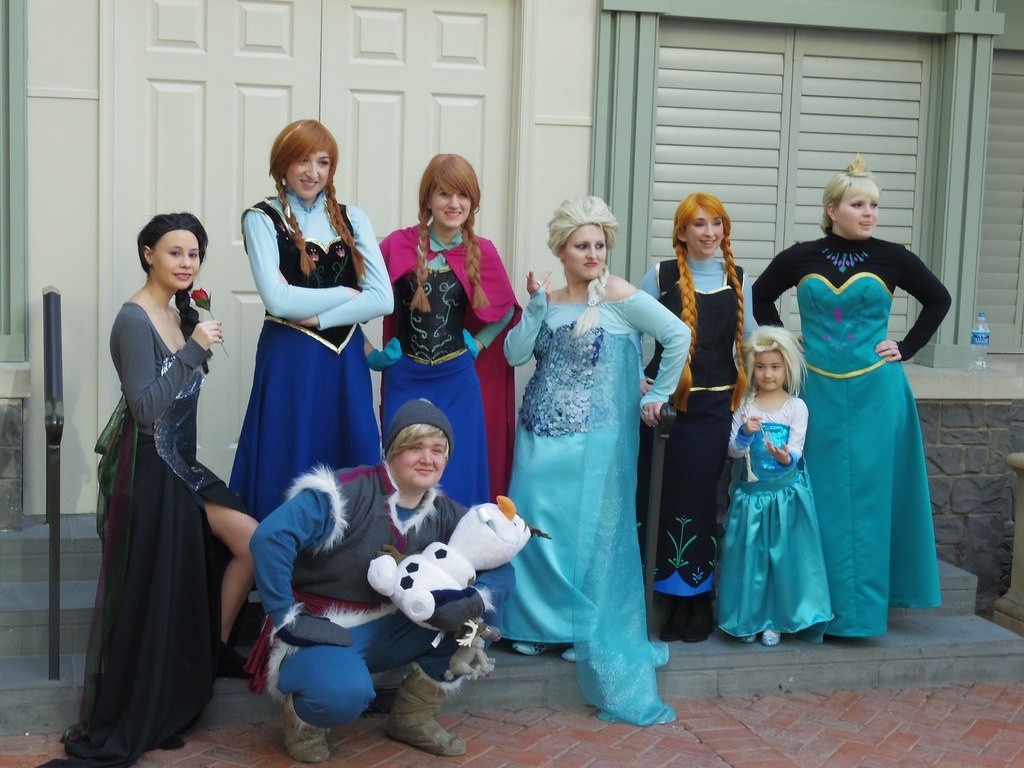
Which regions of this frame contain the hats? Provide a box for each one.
[384,400,454,459]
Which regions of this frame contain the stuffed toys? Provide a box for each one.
[366,490,552,634]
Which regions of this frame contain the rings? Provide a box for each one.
[537,281,541,286]
[644,412,648,416]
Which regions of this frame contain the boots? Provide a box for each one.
[385,663,467,756]
[660,595,713,642]
[283,693,330,763]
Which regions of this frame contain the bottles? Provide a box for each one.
[968,313,991,376]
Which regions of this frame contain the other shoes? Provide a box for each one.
[158,736,185,750]
[216,641,253,680]
[744,630,780,646]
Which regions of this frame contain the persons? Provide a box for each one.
[379,153,522,509]
[225,119,382,522]
[635,192,755,649]
[752,152,952,650]
[38,211,261,768]
[715,327,836,647]
[477,195,694,729]
[248,394,516,762]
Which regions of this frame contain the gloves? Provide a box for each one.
[422,587,486,631]
[274,611,352,647]
[366,337,402,372]
[463,328,478,360]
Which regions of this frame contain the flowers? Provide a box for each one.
[192,288,229,358]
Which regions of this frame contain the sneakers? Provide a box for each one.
[512,642,568,655]
[562,645,590,662]
